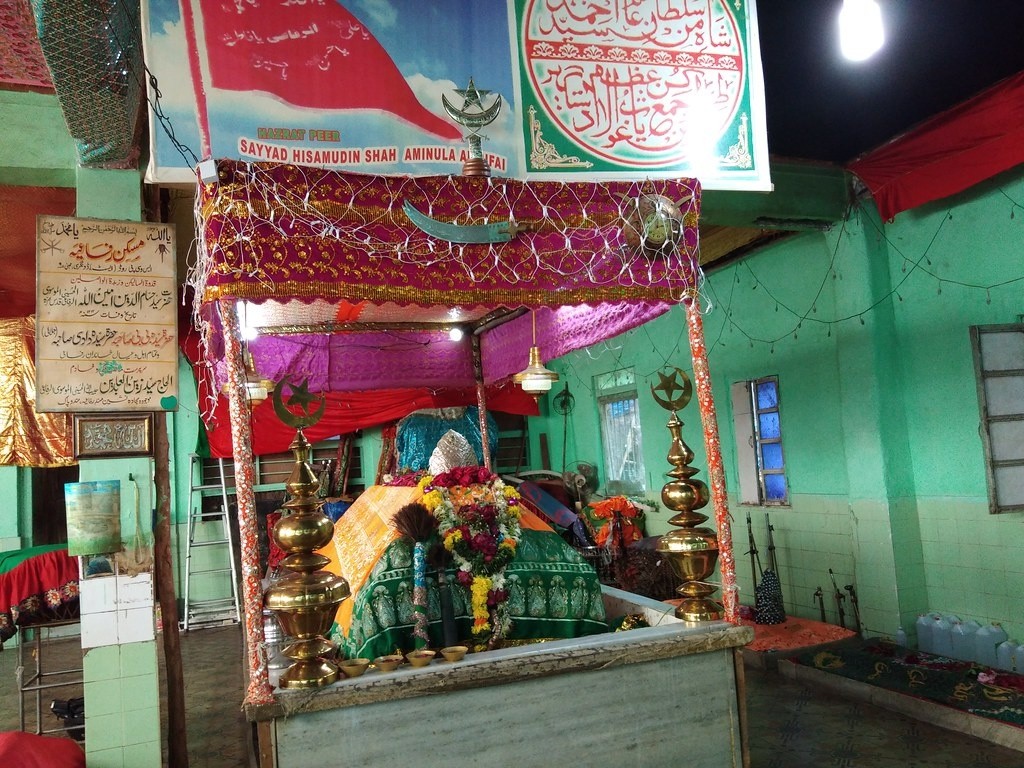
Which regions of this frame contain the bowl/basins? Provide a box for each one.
[373,655,404,672]
[406,650,436,667]
[440,646,468,662]
[338,658,370,677]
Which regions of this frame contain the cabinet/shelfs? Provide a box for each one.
[0,544,85,745]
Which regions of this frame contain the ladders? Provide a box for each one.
[181,452,242,636]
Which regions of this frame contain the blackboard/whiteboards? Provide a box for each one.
[35,212,180,413]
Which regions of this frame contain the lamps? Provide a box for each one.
[220,301,274,406]
[512,310,560,394]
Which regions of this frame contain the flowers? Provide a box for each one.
[426,465,522,653]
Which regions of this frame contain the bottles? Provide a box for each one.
[897,625,906,647]
[916,611,1024,675]
